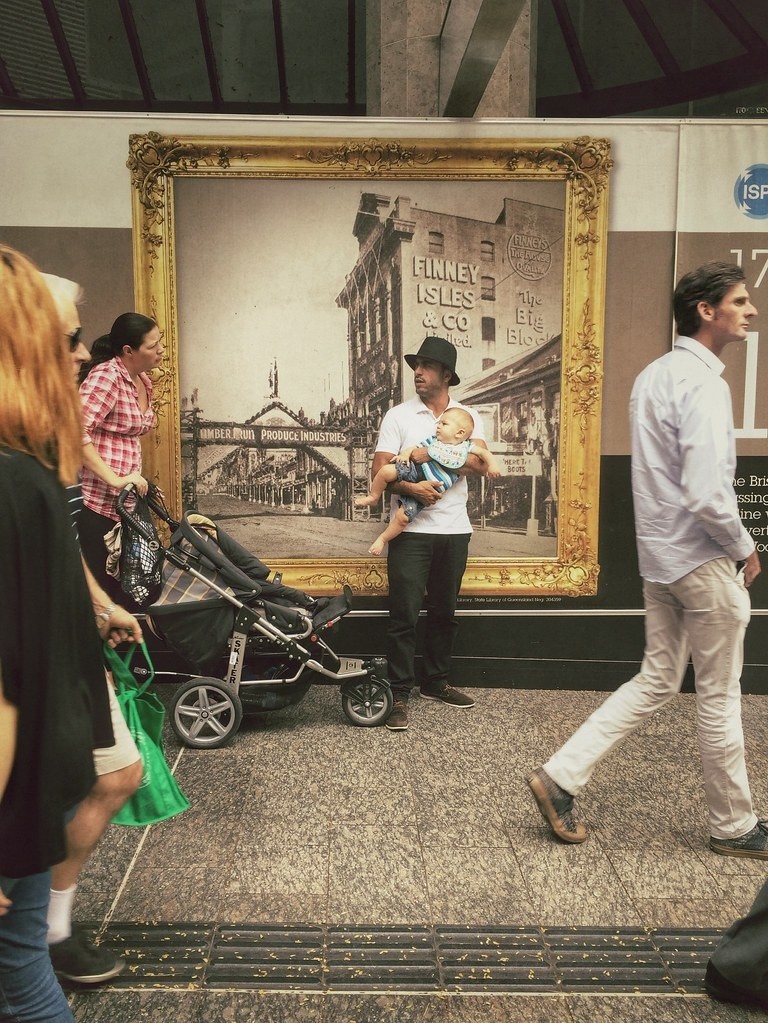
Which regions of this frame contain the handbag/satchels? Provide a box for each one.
[103,628,189,826]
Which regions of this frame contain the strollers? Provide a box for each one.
[110,481,394,750]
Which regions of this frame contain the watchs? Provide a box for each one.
[95,602,116,628]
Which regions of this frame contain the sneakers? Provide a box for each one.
[420,683,475,708]
[524,768,588,843]
[385,698,409,729]
[708,819,768,860]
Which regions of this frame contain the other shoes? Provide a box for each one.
[48,933,122,980]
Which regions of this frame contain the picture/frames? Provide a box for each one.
[125,130,615,614]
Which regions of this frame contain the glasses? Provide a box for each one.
[63,327,82,352]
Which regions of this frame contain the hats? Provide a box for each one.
[404,337,460,385]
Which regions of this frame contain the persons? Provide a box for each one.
[78,313,165,695]
[0,241,117,1023]
[356,407,502,557]
[523,260,768,862]
[371,336,489,729]
[705,877,768,1008]
[41,271,144,983]
